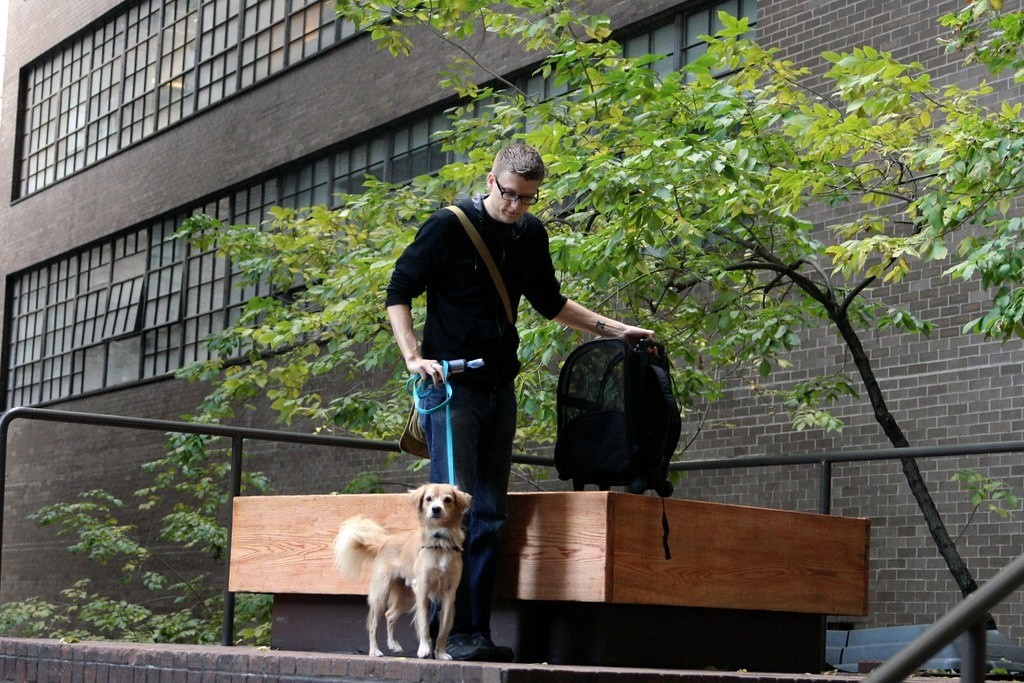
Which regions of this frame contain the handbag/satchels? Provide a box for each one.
[397,400,430,459]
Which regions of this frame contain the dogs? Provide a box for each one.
[330,479,475,662]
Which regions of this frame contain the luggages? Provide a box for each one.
[552,333,681,497]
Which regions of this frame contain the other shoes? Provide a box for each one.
[439,637,515,661]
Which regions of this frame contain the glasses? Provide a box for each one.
[494,175,540,205]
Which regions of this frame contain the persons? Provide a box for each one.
[384,144,656,660]
